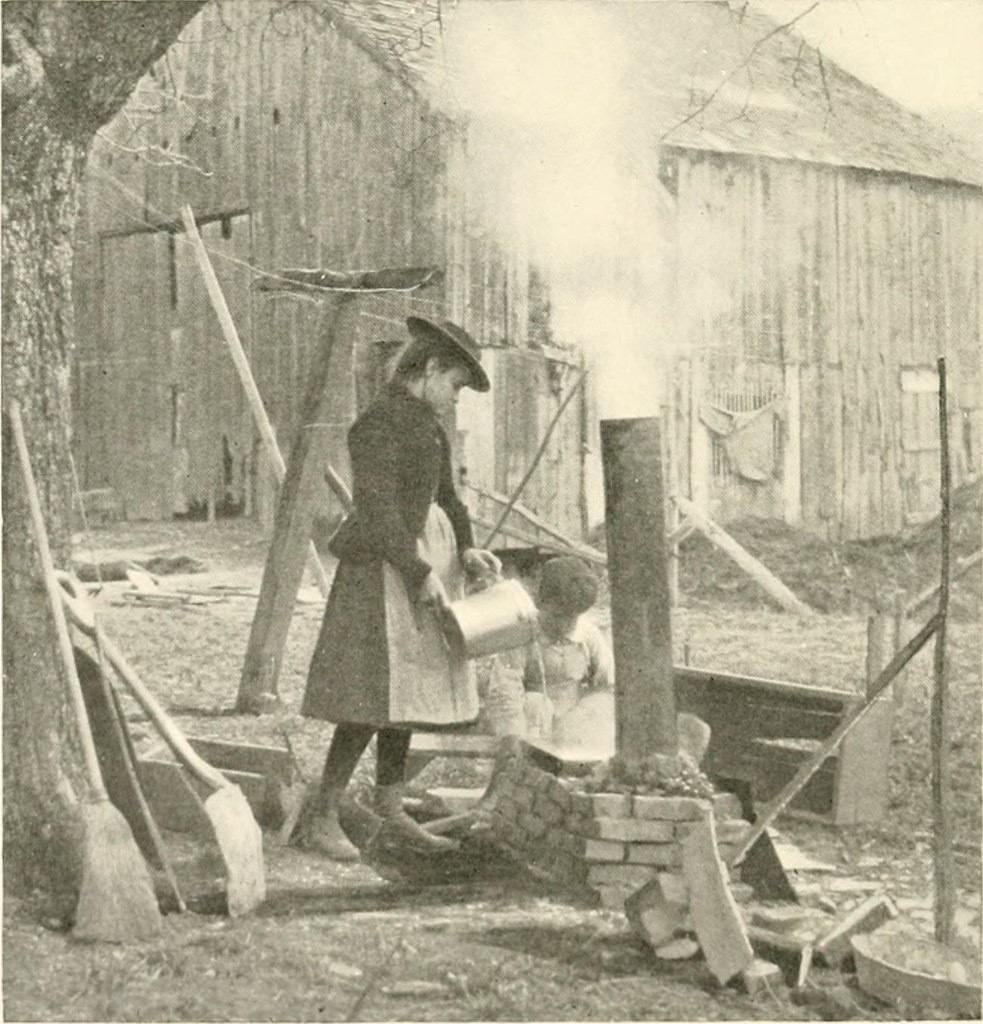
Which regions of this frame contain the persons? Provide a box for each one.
[488,555,608,755]
[300,318,501,857]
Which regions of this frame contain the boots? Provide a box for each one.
[371,782,461,855]
[302,781,360,859]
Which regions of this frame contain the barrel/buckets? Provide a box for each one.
[442,570,540,659]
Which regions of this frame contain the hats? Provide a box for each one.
[407,313,491,393]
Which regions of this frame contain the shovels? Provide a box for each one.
[51,570,268,919]
